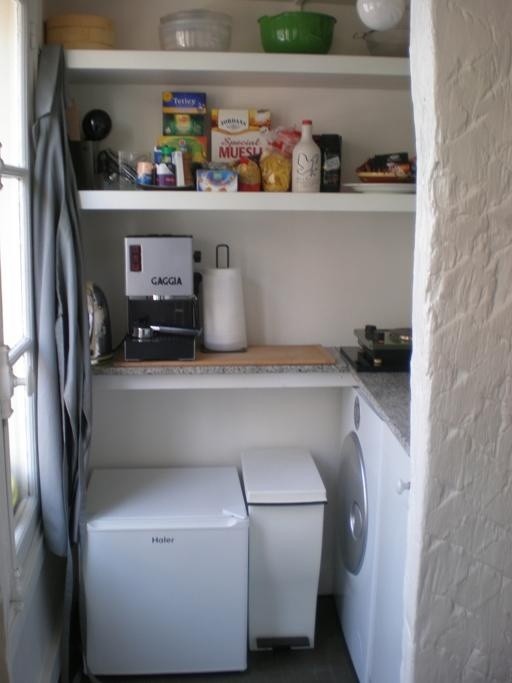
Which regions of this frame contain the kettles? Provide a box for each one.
[86,280,115,366]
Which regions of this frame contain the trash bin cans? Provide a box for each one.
[241,449,327,653]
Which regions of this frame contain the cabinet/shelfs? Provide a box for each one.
[32,49,417,212]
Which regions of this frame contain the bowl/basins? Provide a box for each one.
[159,8,231,50]
[45,13,115,49]
[353,27,410,56]
[259,11,338,53]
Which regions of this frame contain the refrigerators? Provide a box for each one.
[86,468,245,672]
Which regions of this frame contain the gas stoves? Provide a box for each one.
[354,324,411,364]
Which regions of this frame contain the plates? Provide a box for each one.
[355,169,415,182]
[343,181,416,193]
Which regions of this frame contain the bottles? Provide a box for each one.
[153,139,195,187]
[291,119,321,193]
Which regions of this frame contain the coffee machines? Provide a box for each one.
[122,235,197,359]
